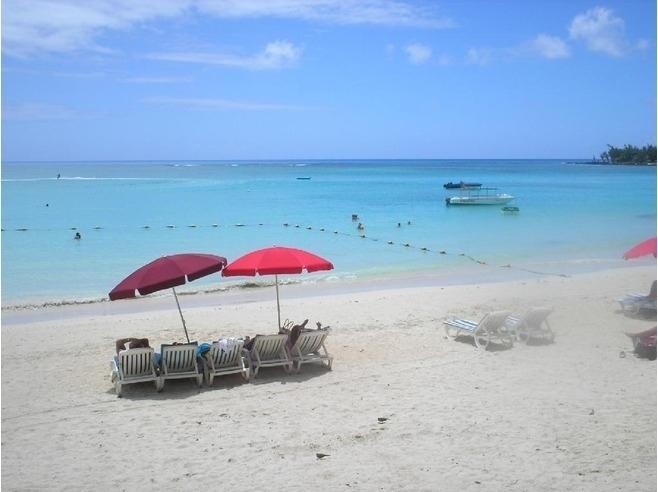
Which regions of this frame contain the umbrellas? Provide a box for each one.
[621,236,657,261]
[220,244,336,335]
[108,253,228,343]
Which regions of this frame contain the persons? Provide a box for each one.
[115,337,150,355]
[356,222,365,230]
[75,232,82,238]
[396,223,402,227]
[244,318,322,352]
[407,221,411,226]
[631,279,656,315]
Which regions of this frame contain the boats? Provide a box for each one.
[443,180,522,215]
[295,176,311,180]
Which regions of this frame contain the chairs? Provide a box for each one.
[444,310,556,350]
[616,292,657,316]
[111,330,334,398]
